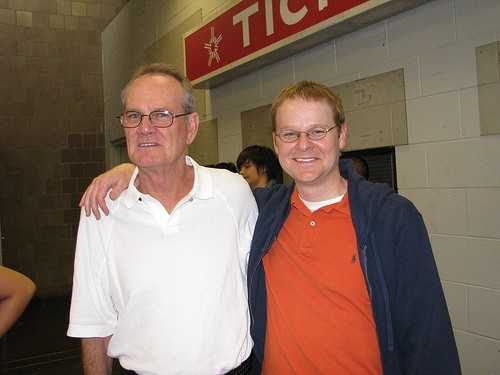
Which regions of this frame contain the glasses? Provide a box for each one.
[117,110,192,129]
[274,125,339,143]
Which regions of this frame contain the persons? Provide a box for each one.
[0,265,37,340]
[237,145,283,188]
[337,155,369,182]
[77,79,462,374]
[66,61,260,374]
